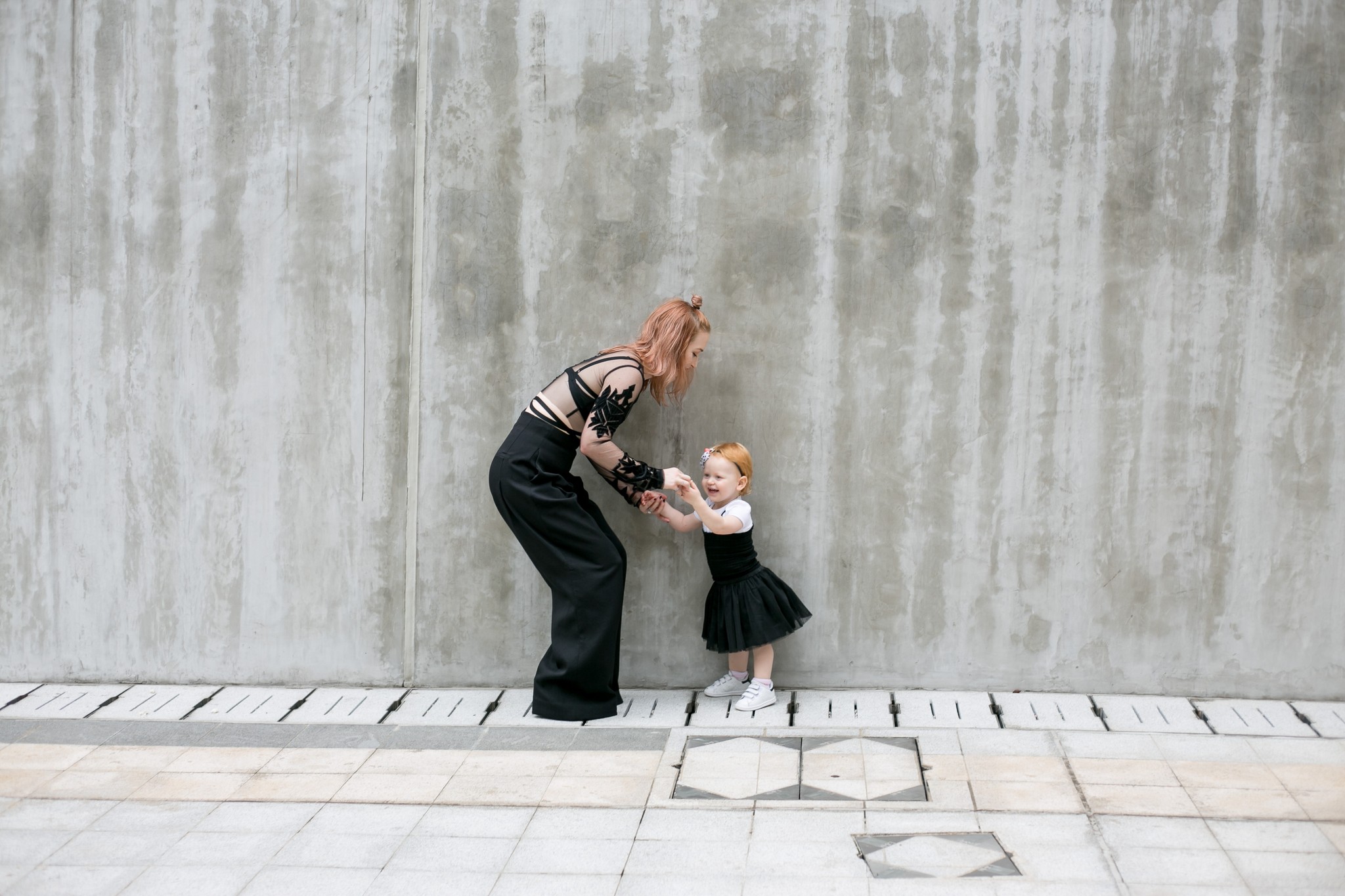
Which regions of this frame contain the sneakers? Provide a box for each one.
[704,670,751,697]
[735,678,777,711]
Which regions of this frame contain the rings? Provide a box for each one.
[647,508,652,513]
[681,479,685,485]
[674,487,680,491]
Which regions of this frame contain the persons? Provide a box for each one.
[488,295,711,721]
[640,442,813,712]
